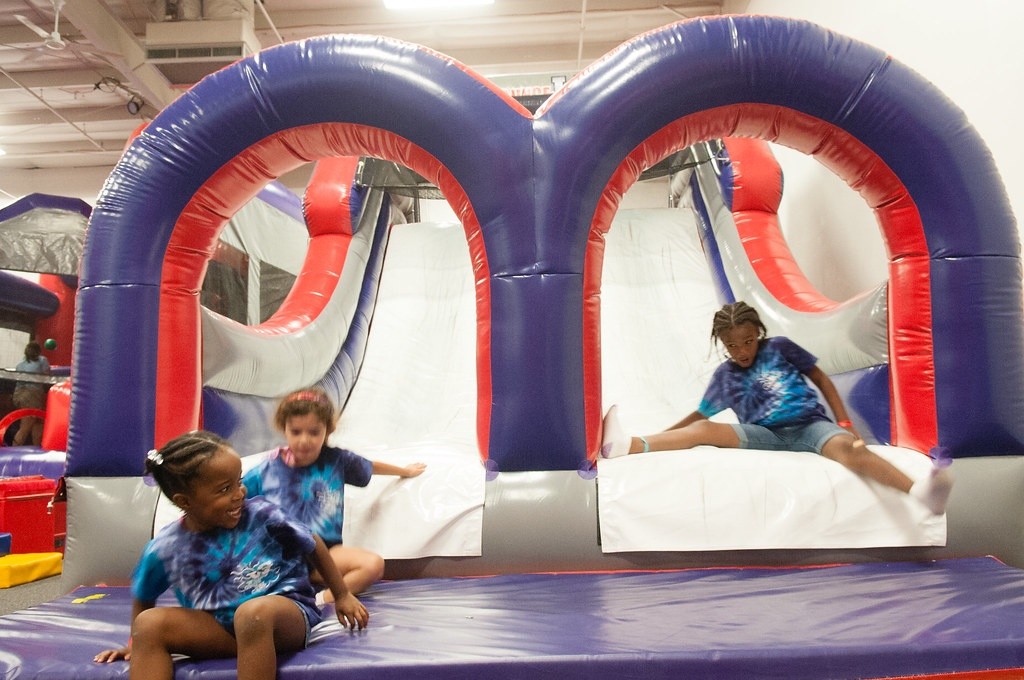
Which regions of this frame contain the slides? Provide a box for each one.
[141,138,936,561]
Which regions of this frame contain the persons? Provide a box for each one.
[239,388,428,612]
[93,430,370,680]
[11,341,50,447]
[601,301,955,516]
